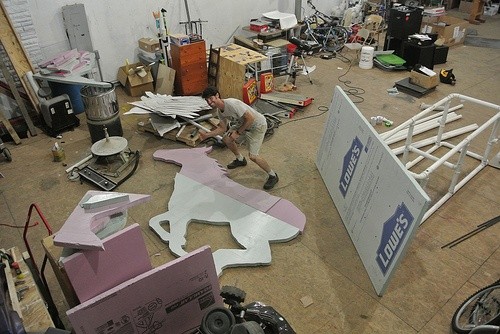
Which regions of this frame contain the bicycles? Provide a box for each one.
[451,277,500,334]
[300,0,350,55]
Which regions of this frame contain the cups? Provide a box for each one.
[370,116,383,126]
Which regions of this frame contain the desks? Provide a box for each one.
[211,43,268,102]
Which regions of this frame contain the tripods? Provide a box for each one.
[285,54,313,86]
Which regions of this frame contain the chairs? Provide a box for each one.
[342,14,383,66]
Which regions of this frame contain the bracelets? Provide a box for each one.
[236,130,241,135]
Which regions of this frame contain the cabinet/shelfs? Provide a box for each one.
[242,24,289,82]
[171,40,209,95]
[384,7,424,57]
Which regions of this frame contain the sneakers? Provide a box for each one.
[263,173,279,190]
[227,157,247,169]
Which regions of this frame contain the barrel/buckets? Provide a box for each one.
[51,146,65,162]
[359,46,374,69]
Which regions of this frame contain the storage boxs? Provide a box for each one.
[138,54,160,78]
[117,63,155,97]
[259,73,273,94]
[424,6,446,17]
[242,78,258,106]
[138,38,159,52]
[250,22,268,33]
[408,69,439,89]
[401,16,469,71]
[458,1,474,13]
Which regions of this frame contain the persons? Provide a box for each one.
[198,86,279,190]
